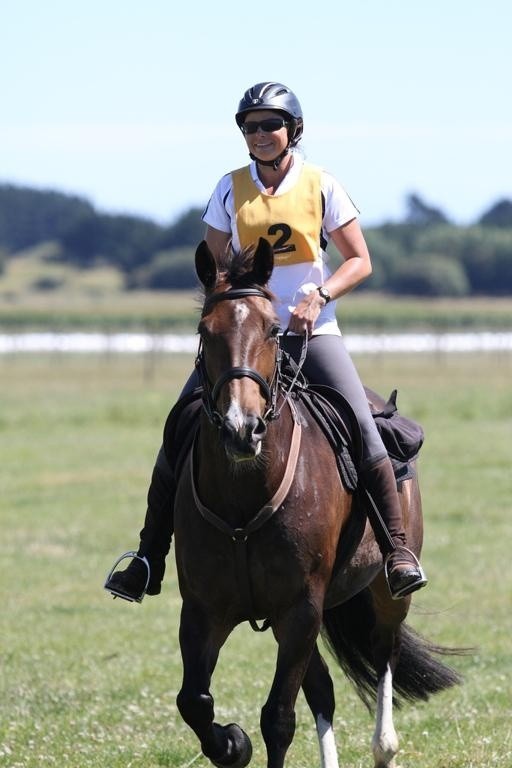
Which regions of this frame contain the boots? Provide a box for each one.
[358,456,422,598]
[106,490,173,600]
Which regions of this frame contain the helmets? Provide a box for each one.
[234,81,304,148]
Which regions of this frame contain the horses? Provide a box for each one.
[174,237,478,768]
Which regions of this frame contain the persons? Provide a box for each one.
[104,82,427,599]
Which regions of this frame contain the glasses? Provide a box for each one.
[242,118,289,134]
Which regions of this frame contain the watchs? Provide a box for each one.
[317,287,331,304]
